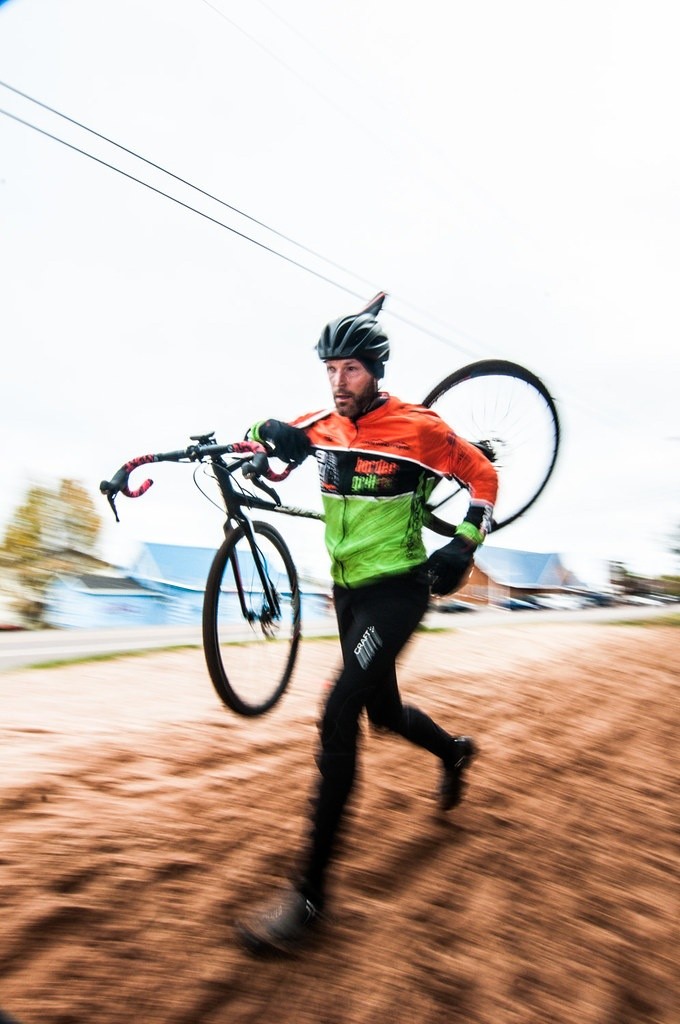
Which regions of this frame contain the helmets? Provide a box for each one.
[318,311,391,362]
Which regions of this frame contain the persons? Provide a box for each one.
[229,310,504,962]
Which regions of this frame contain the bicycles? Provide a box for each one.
[104,359,567,722]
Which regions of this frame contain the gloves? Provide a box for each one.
[424,532,477,598]
[245,418,310,466]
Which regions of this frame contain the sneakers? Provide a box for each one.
[442,736,479,812]
[278,881,326,944]
